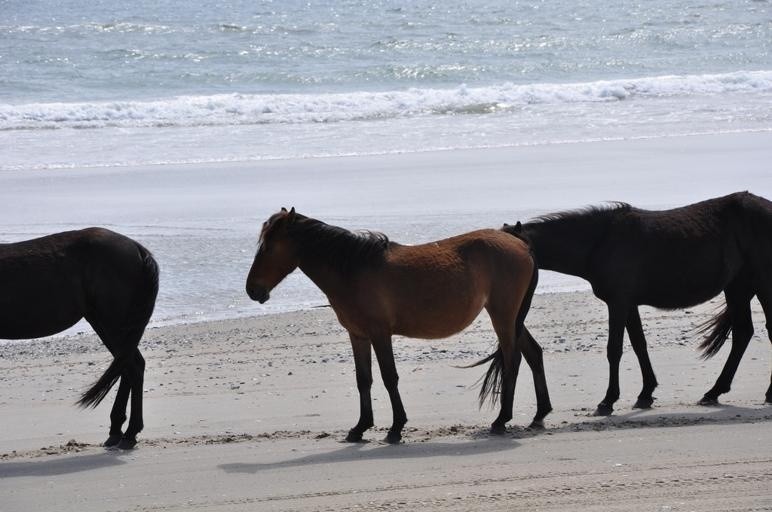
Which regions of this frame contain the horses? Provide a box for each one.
[0,227,160,450]
[245,206,552,445]
[499,189,772,416]
